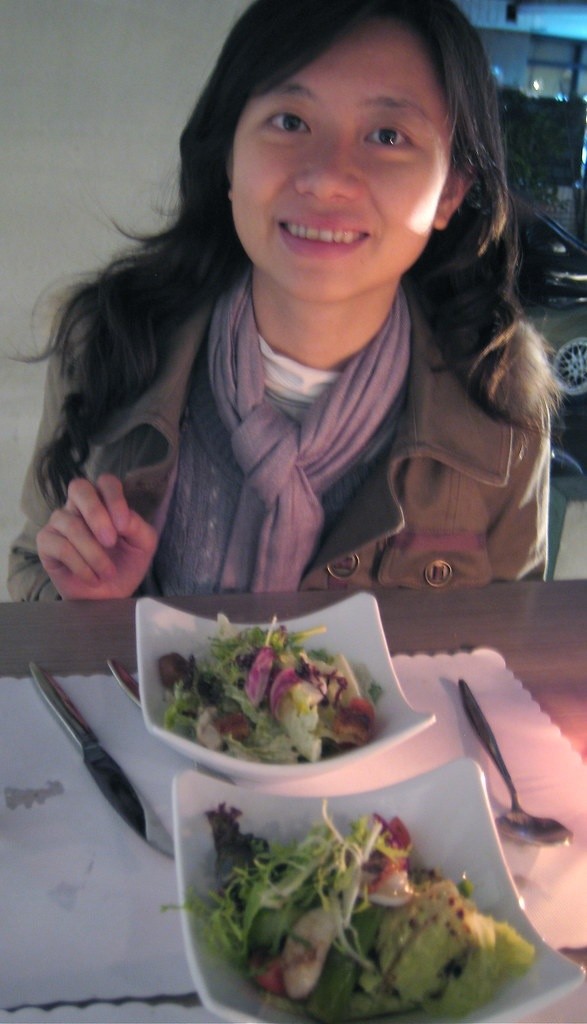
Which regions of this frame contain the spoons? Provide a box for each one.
[456,676,574,846]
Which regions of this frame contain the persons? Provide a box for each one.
[7,1,557,605]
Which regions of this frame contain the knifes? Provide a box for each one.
[31,661,153,845]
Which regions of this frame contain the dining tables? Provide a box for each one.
[0,576,587,1024]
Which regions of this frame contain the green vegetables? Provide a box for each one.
[161,614,387,767]
[159,797,537,1024]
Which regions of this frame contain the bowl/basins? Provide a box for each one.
[134,593,436,782]
[169,762,585,1024]
